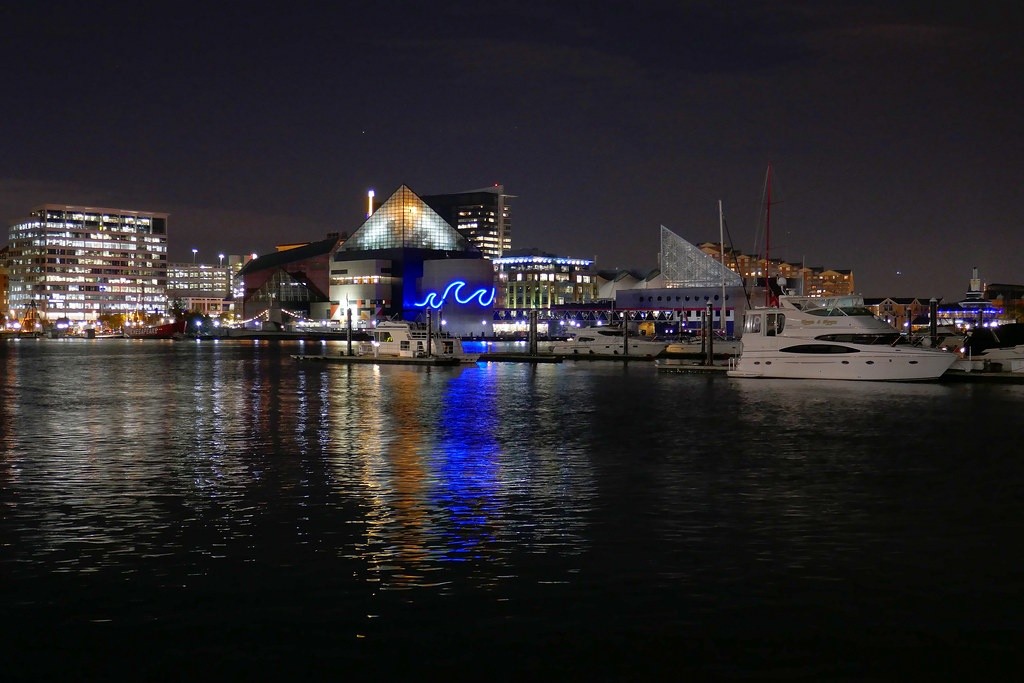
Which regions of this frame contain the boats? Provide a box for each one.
[123,324,174,338]
[291,319,482,367]
[726,166,960,381]
[947,322,1024,376]
[524,326,671,358]
[655,200,743,355]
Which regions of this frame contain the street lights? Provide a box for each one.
[706,300,714,367]
[196,320,202,333]
[426,306,431,359]
[905,307,912,342]
[437,309,442,333]
[528,309,537,356]
[368,190,375,217]
[219,254,225,264]
[623,310,628,354]
[98,287,107,316]
[928,296,939,346]
[346,306,352,356]
[5,315,8,330]
[191,248,198,264]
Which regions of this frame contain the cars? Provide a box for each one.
[56,317,73,329]
[102,329,112,335]
